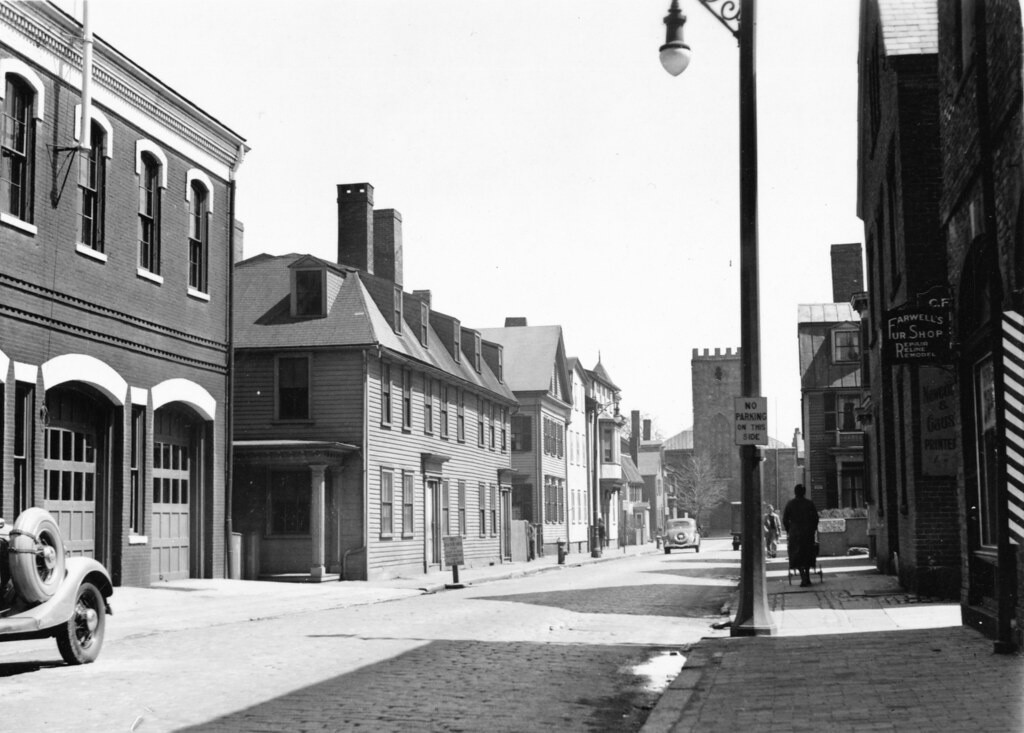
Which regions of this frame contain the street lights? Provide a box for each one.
[655,0,782,638]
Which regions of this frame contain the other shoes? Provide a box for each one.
[800,582,811,587]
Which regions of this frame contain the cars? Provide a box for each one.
[661,517,701,554]
[0,507,112,665]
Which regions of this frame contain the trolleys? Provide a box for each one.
[786,528,824,586]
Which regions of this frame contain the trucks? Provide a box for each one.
[728,499,771,551]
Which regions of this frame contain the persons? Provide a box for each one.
[865,498,877,559]
[763,504,782,558]
[599,518,606,554]
[782,483,819,587]
